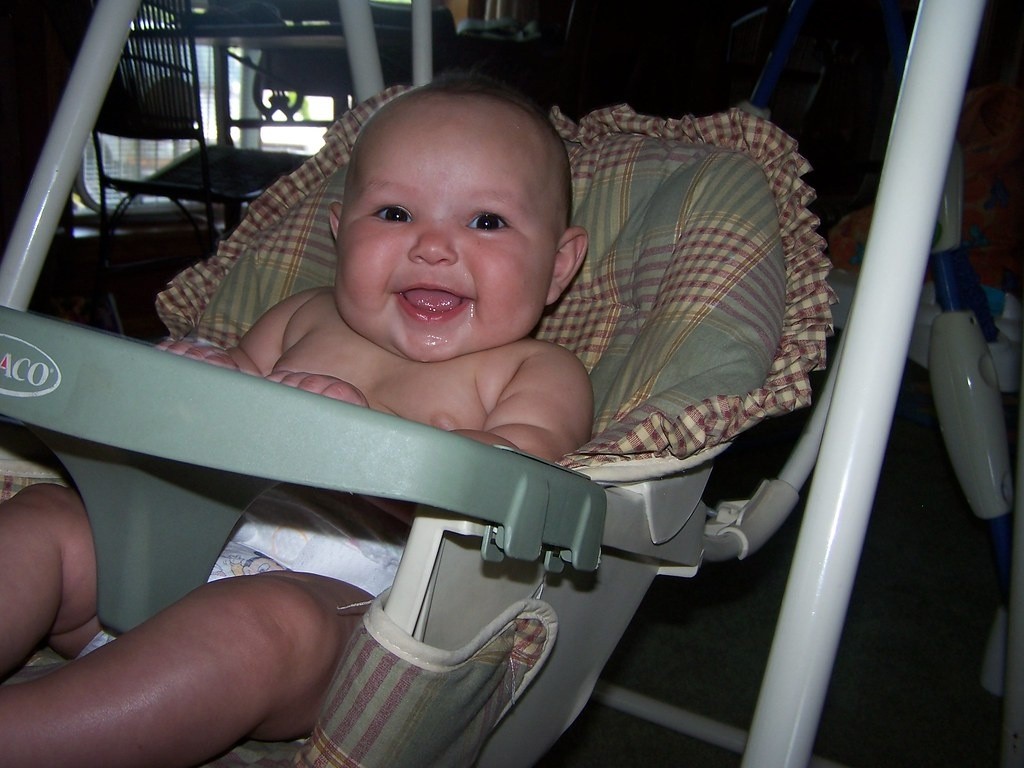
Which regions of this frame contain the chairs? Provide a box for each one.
[68,0,316,344]
[1,70,839,768]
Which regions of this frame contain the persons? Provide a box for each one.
[0,76,596,768]
